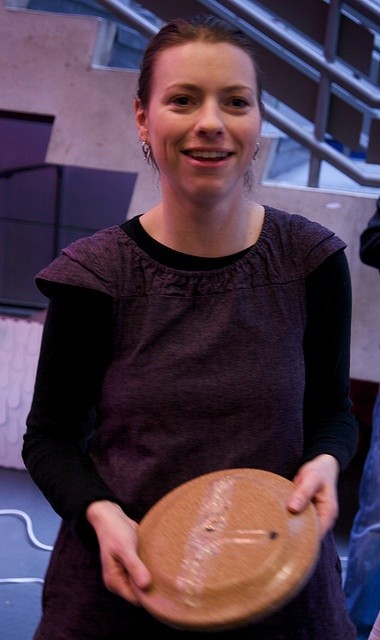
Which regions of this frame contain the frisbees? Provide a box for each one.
[126,468,321,631]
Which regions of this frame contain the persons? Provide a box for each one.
[19,15,361,640]
[342,191,380,639]
